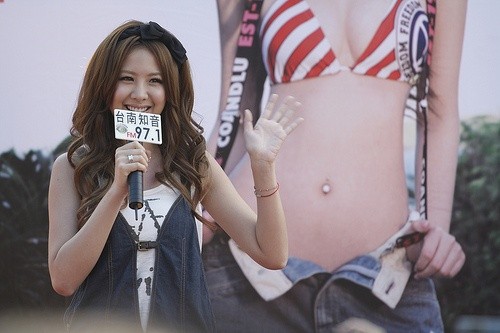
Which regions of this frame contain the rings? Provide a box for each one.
[127,154,134,162]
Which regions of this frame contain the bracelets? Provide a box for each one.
[253,183,279,198]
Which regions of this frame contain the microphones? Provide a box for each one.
[114,109,162,211]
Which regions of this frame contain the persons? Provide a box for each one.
[202,0,469,333]
[48,20,304,333]
